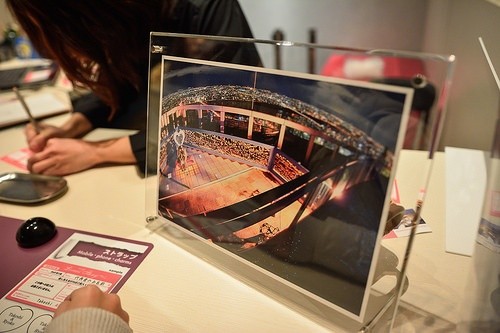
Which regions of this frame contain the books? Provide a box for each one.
[0,85,71,129]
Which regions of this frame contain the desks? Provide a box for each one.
[1,121,500,333]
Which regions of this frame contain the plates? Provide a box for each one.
[0,171,67,204]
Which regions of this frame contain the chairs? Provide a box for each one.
[374,76,436,151]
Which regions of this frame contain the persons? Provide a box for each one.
[5,0,266,176]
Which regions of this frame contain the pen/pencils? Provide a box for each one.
[13,87,40,135]
